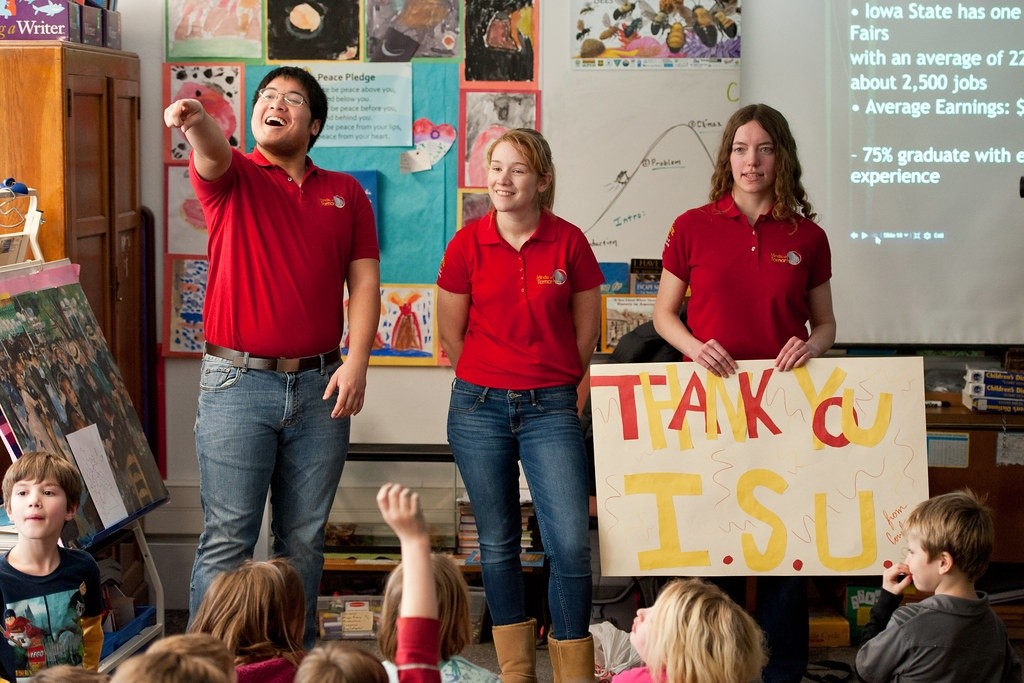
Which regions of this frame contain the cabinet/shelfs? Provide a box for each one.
[924,409,1023,594]
[0,40,145,448]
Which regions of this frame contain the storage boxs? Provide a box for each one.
[318,596,384,643]
[0,0,121,50]
[809,586,882,647]
[320,444,459,553]
[465,585,484,647]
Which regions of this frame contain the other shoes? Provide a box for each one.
[763,663,803,683]
[302,631,316,650]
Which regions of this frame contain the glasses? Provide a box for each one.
[257,87,312,107]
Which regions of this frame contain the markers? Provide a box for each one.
[925,400,951,409]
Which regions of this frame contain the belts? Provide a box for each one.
[205,341,342,374]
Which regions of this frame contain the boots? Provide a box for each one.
[492,617,538,683]
[547,631,595,683]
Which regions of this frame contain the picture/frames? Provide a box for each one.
[601,294,657,352]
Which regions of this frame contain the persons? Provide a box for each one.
[436,128,606,683]
[28,633,237,683]
[188,559,311,683]
[163,67,381,652]
[378,553,502,683]
[294,482,436,683]
[652,103,837,683]
[612,578,765,683]
[854,492,1024,683]
[0,453,105,683]
